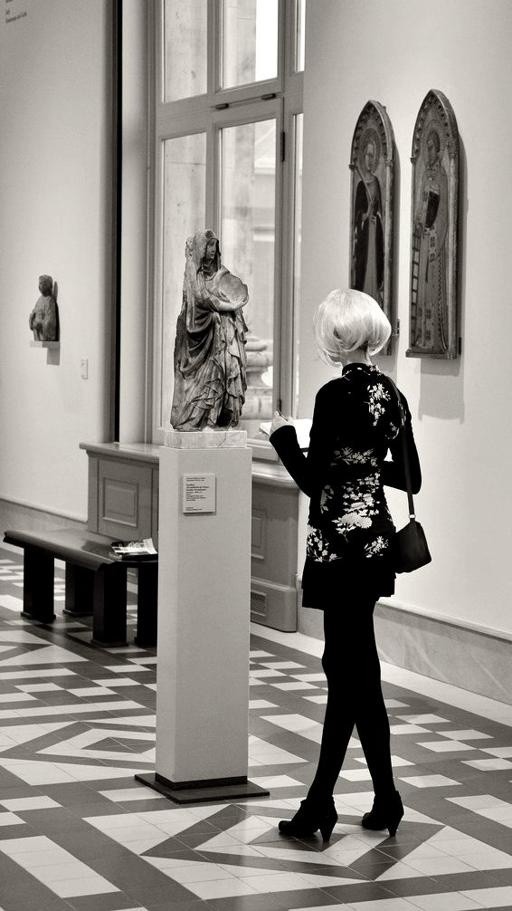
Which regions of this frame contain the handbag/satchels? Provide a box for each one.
[393,521,432,574]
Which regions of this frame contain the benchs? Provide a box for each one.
[3,528,158,648]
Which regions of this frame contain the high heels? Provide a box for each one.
[278,799,338,843]
[361,793,406,836]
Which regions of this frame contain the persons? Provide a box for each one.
[29,274,60,341]
[170,228,248,432]
[268,289,422,844]
[413,129,449,354]
[353,140,384,308]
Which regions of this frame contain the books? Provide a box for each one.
[108,537,158,563]
